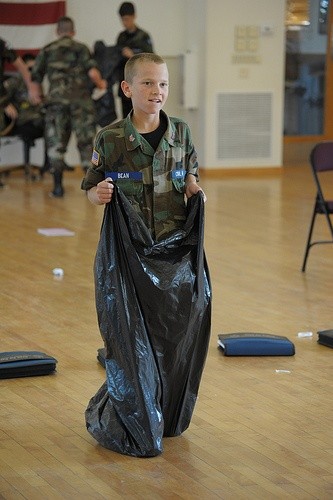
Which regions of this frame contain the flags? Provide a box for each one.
[0,0,67,72]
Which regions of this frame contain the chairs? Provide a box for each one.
[5,72,48,181]
[300,141,333,272]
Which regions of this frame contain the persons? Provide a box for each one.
[0,3,158,198]
[80,55,208,240]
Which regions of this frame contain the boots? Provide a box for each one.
[53,172,64,196]
